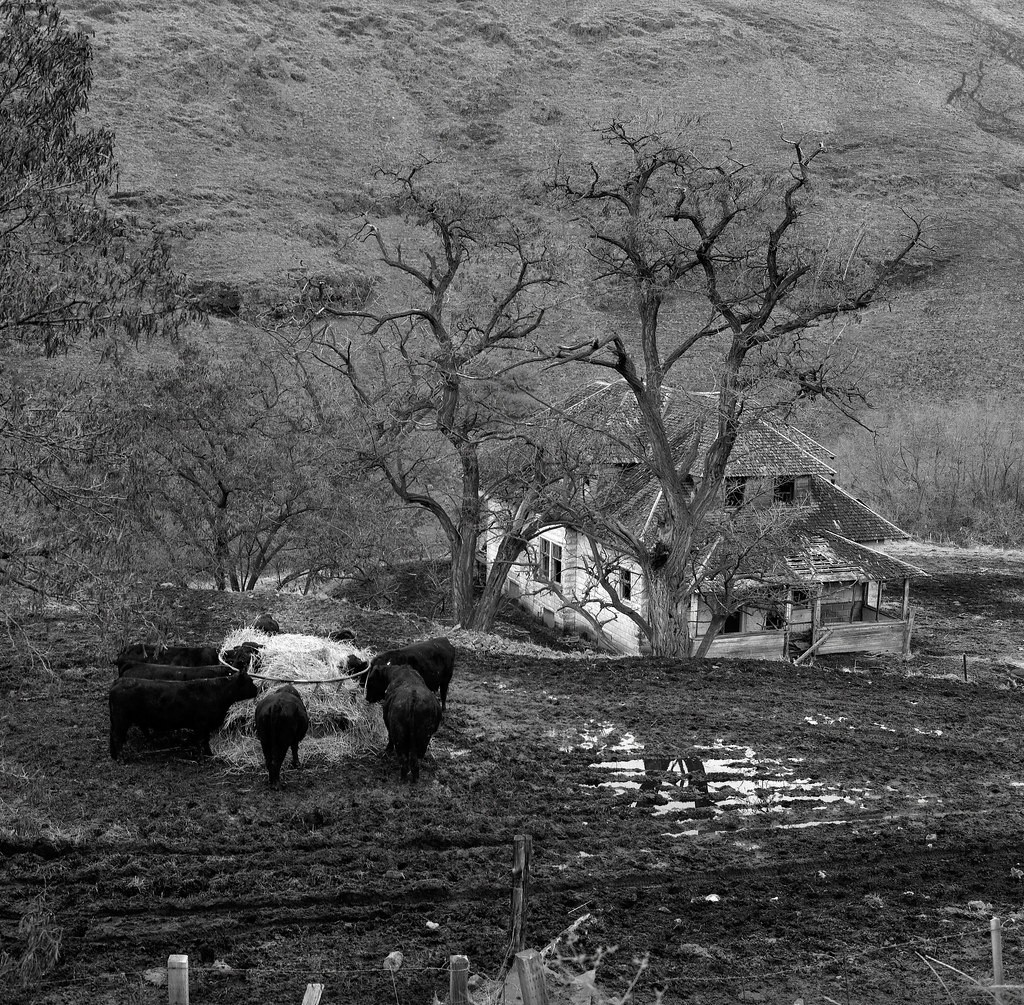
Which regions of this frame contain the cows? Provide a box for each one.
[252,611,369,689]
[365,636,457,711]
[365,663,442,785]
[255,684,310,790]
[109,640,268,762]
